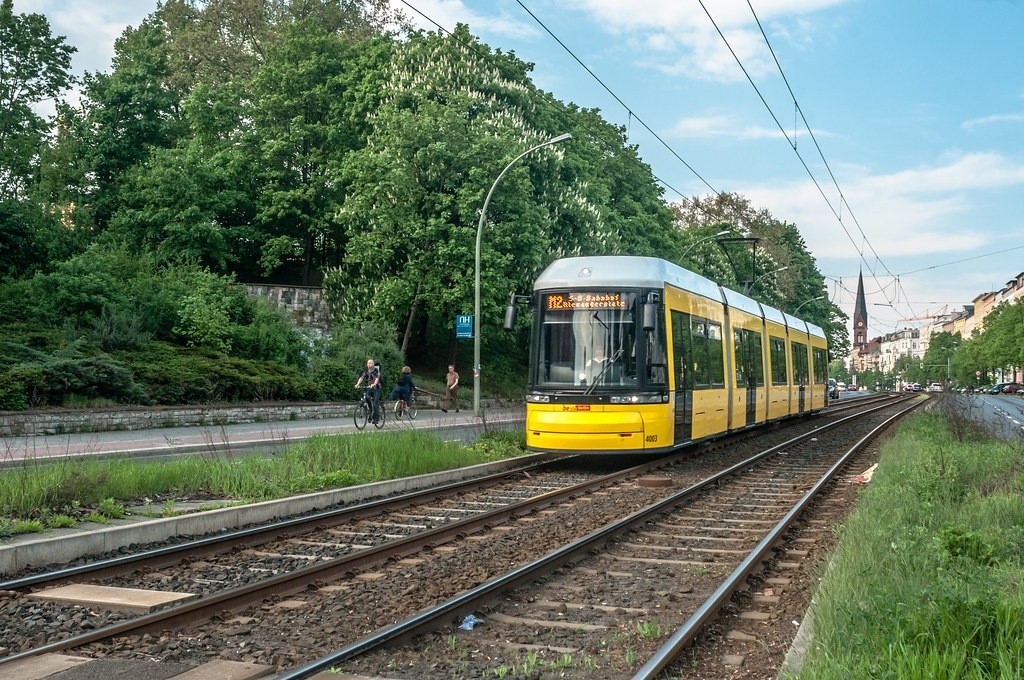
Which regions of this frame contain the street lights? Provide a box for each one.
[471,132,576,413]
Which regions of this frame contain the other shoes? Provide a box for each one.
[456,409,459,413]
[372,420,378,424]
[441,408,447,413]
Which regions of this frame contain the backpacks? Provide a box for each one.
[397,373,410,386]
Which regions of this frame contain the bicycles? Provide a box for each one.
[391,387,418,420]
[354,385,387,430]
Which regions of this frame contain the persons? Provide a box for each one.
[579,342,612,384]
[945,378,957,393]
[440,365,460,413]
[389,365,418,416]
[833,385,839,399]
[353,359,383,424]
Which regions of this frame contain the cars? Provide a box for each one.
[837,379,1024,396]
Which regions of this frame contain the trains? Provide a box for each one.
[504,254,831,457]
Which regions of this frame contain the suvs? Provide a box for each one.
[828,378,840,398]
